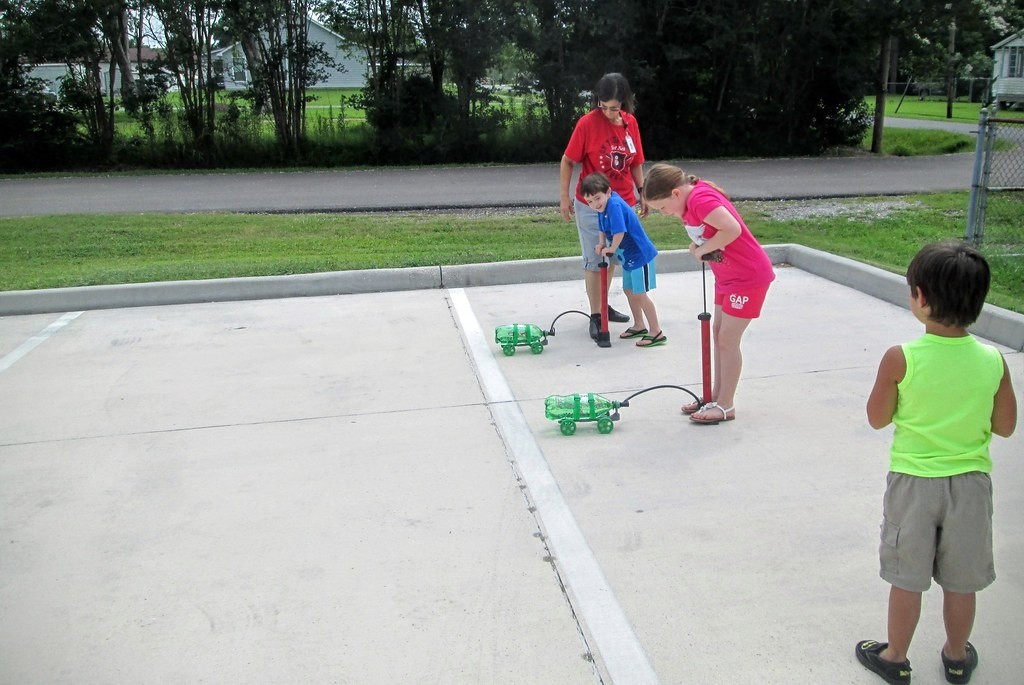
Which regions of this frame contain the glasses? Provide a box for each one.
[598,99,621,111]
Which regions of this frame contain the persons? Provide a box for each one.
[580,173,666,347]
[854,238,1018,685]
[643,163,776,423]
[560,73,644,340]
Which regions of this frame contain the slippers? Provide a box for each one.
[636,331,667,348]
[620,328,648,339]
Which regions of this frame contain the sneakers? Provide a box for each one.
[608,304,630,322]
[589,316,601,338]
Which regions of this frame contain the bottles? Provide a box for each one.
[546,393,621,421]
[495,324,548,343]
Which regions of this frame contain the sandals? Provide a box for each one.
[682,396,706,413]
[689,402,735,423]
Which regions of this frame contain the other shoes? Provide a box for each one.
[855,639,912,685]
[942,641,978,685]
